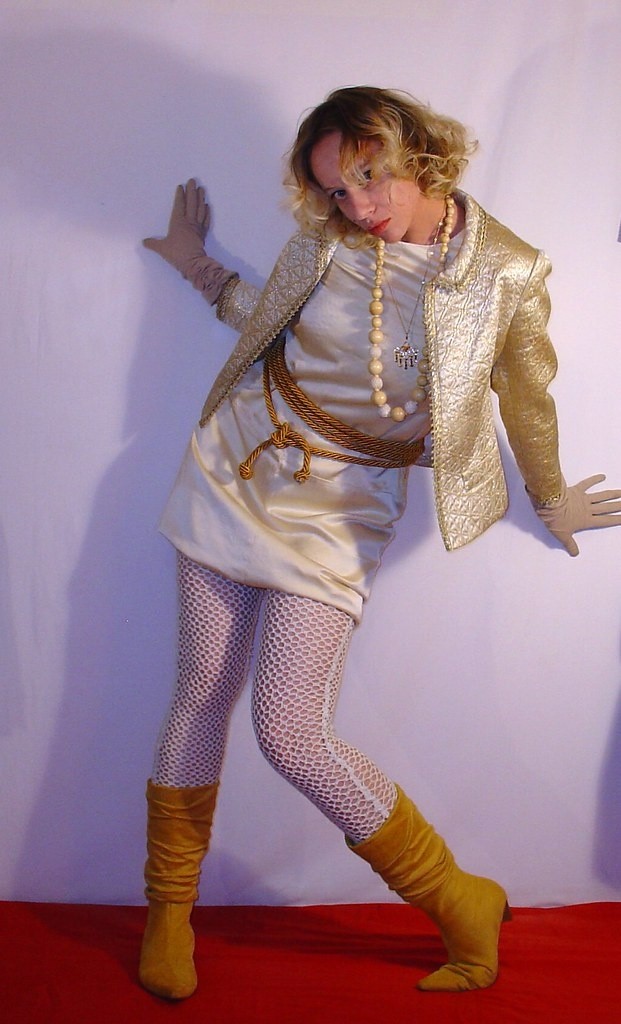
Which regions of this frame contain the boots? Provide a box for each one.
[345,780,513,991]
[138,777,220,998]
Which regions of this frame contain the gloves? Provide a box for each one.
[143,178,240,306]
[524,474,621,557]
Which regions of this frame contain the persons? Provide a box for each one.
[136,85,620,1005]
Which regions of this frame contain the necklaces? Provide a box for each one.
[382,202,447,370]
[369,195,455,422]
